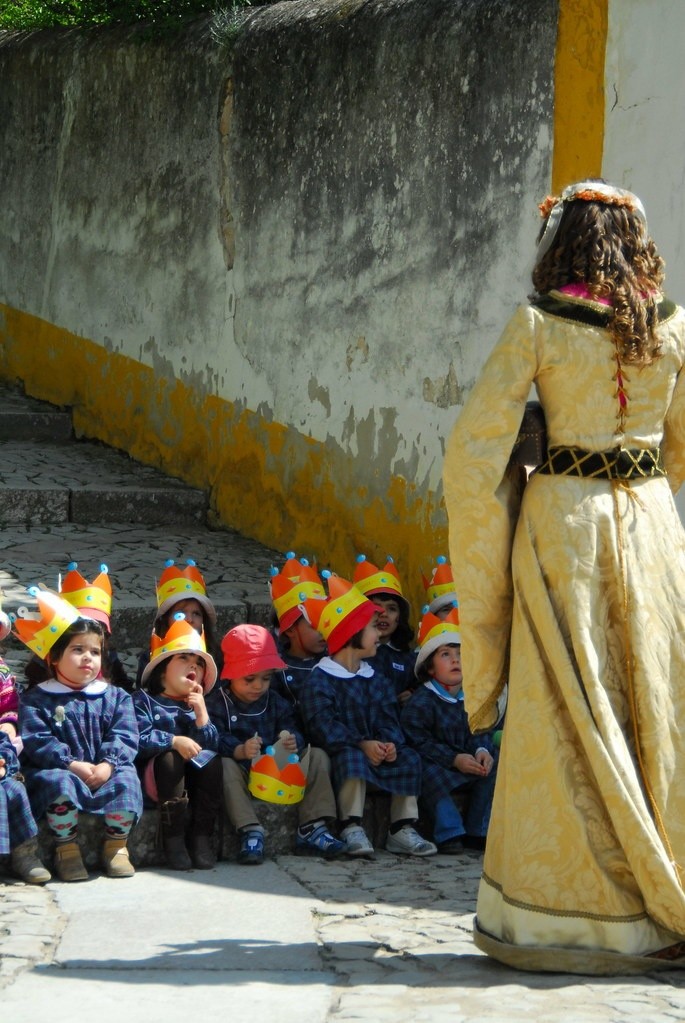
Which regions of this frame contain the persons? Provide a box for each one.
[443,181,685,974]
[132,553,531,873]
[1,562,143,886]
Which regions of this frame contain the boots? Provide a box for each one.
[52,841,88,881]
[155,788,192,869]
[11,837,52,884]
[101,839,136,876]
[187,786,225,869]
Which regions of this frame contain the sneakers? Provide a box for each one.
[386,827,437,855]
[339,825,373,854]
[296,820,347,859]
[235,825,265,862]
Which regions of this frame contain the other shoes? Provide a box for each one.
[439,834,464,853]
[461,833,487,850]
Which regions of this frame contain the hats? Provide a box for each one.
[279,603,305,637]
[141,648,217,699]
[363,588,410,623]
[414,633,461,677]
[327,601,384,655]
[153,592,217,632]
[219,625,287,681]
[0,609,12,641]
[78,608,111,630]
[428,593,458,616]
